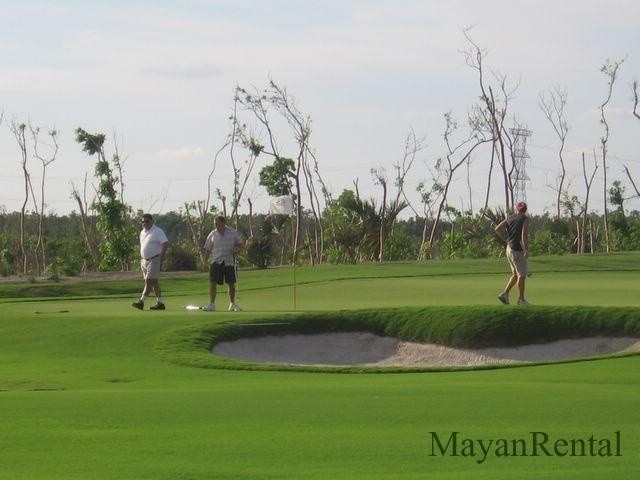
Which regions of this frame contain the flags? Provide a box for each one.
[270,193,294,214]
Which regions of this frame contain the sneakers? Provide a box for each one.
[497,292,509,304]
[517,298,529,305]
[132,300,144,309]
[228,304,240,312]
[201,303,216,311]
[150,302,165,310]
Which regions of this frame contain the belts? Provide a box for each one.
[146,255,159,261]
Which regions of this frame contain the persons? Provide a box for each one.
[131,214,169,311]
[203,216,244,312]
[494,203,531,305]
